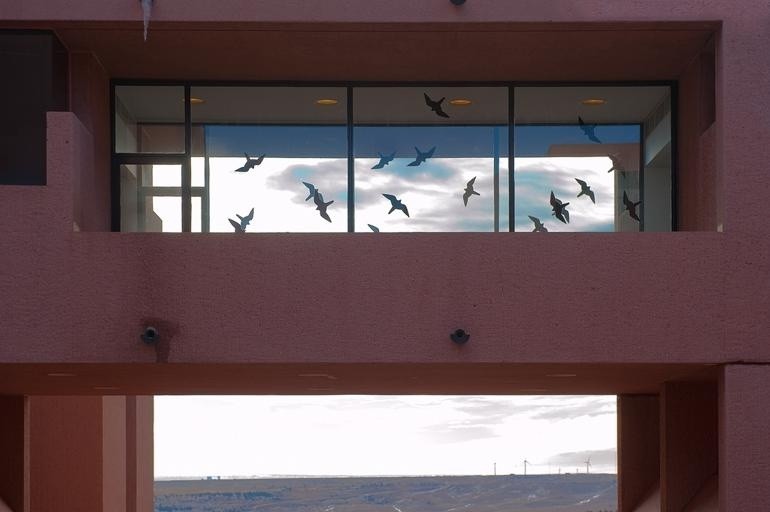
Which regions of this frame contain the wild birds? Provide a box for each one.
[607,153,626,178]
[234,150,267,172]
[228,208,255,233]
[463,176,480,207]
[368,224,381,233]
[302,181,336,223]
[623,189,643,222]
[577,115,605,144]
[527,179,596,232]
[370,145,439,169]
[423,92,451,118]
[382,193,409,218]
